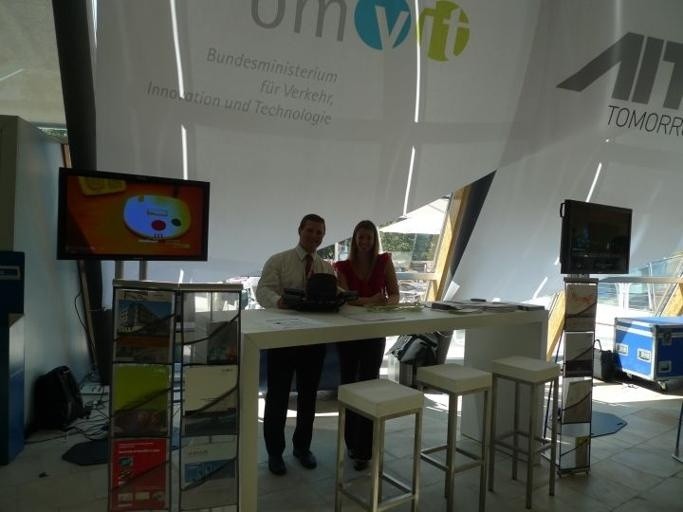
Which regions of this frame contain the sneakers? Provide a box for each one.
[348,448,368,471]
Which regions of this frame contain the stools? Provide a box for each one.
[416,363,493,512]
[335,379,424,512]
[488,356,560,509]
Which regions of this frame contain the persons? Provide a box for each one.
[255,214,338,475]
[333,220,399,472]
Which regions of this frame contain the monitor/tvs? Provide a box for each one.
[559,198,633,275]
[56,166,211,262]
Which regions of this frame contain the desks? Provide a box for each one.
[238,300,548,512]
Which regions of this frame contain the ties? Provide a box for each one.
[304,254,314,280]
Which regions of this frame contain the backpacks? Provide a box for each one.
[35,365,83,428]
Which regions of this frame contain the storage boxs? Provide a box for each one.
[613,317,683,393]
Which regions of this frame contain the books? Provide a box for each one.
[110,288,239,511]
[559,283,598,470]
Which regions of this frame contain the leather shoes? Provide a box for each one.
[295,449,316,469]
[269,456,286,476]
